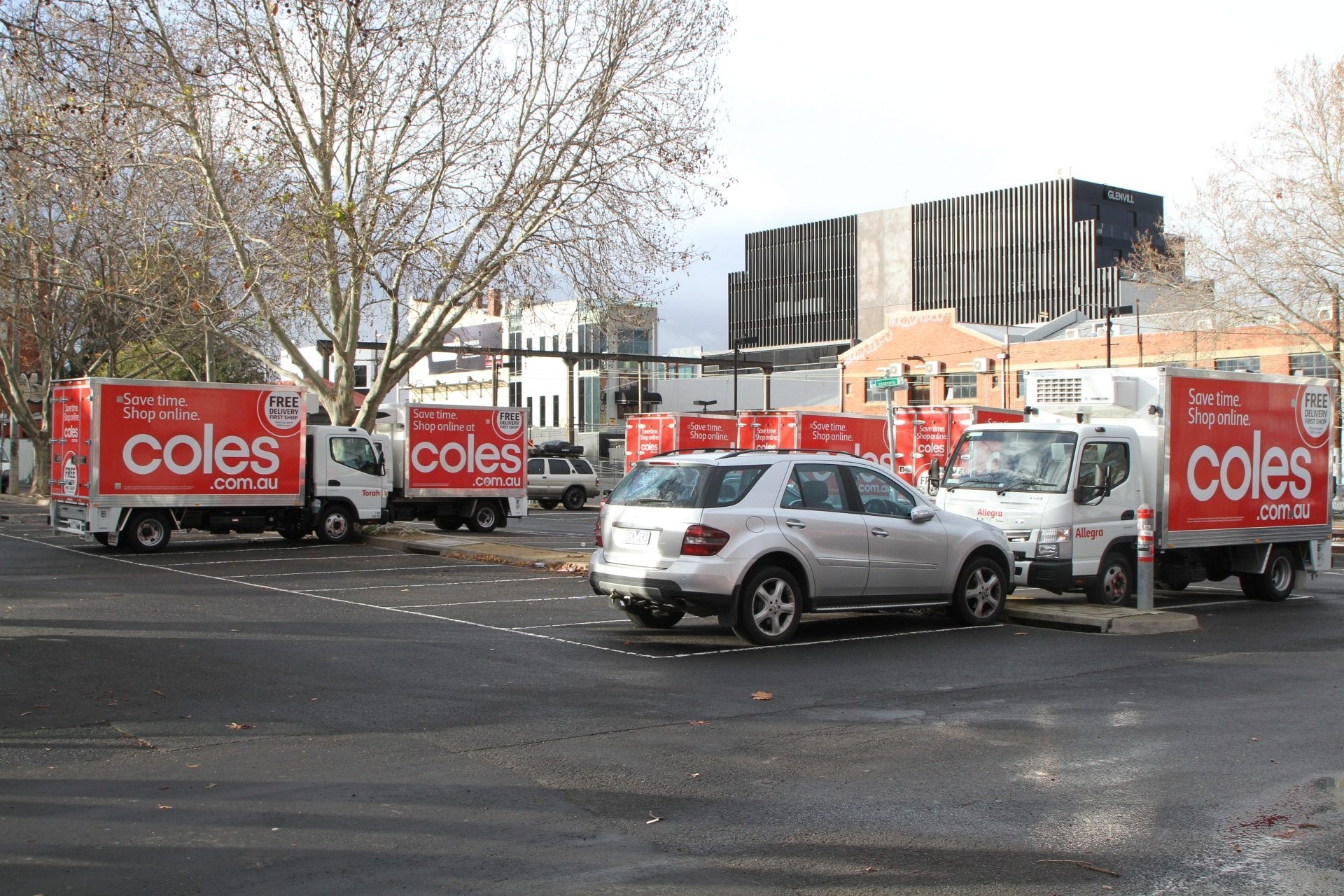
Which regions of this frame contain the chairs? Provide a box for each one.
[790,479,833,509]
[1083,444,1130,495]
[718,484,735,501]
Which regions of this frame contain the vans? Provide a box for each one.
[0,446,10,493]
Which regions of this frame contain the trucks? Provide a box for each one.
[366,404,530,534]
[621,406,1030,497]
[49,377,392,556]
[927,363,1337,604]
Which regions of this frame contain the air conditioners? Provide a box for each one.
[924,361,942,376]
[973,358,991,373]
[890,363,905,377]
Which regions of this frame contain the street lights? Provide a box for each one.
[733,336,760,414]
[693,400,717,414]
[1101,304,1133,368]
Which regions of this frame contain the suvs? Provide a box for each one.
[525,450,601,510]
[583,445,1017,646]
